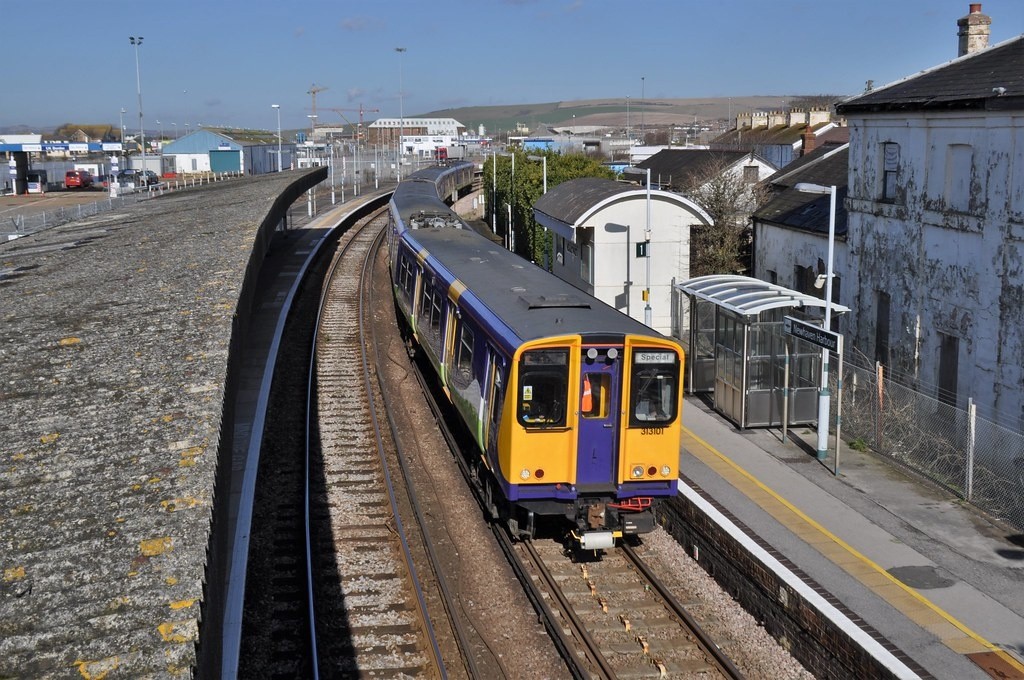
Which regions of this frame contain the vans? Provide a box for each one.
[64,170,94,188]
[117,169,140,180]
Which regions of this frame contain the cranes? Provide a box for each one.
[304,103,379,137]
[304,83,330,140]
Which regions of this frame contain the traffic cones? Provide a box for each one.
[41,191,45,197]
[25,190,29,197]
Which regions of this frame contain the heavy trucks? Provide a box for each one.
[434,146,464,164]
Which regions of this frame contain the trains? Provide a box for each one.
[386,160,685,553]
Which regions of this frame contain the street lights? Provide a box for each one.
[129,35,148,187]
[620,166,651,325]
[796,181,839,460]
[528,156,549,272]
[641,76,646,142]
[153,119,190,142]
[484,150,497,235]
[395,47,408,182]
[272,105,282,172]
[120,108,127,144]
[356,124,362,157]
[500,152,515,252]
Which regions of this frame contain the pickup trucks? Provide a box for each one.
[137,170,158,184]
